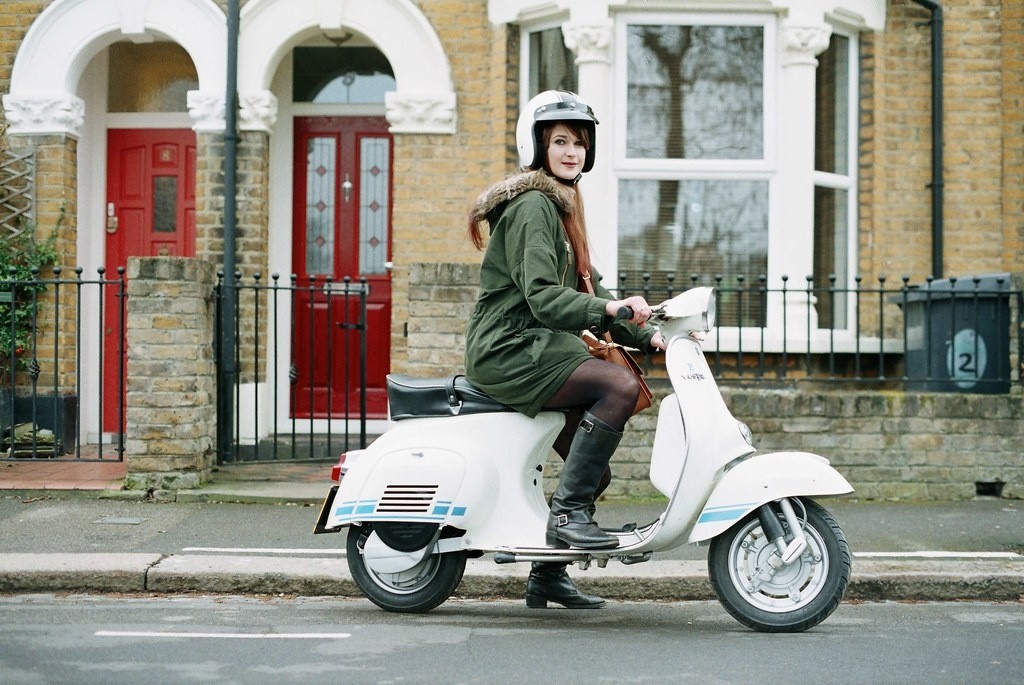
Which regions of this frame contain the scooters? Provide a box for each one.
[313,285,858,633]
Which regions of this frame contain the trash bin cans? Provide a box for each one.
[888,273,1012,392]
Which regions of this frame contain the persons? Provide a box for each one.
[464,90,703,610]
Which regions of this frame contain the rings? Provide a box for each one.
[641,308,645,311]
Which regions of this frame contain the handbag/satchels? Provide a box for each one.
[582,329,653,418]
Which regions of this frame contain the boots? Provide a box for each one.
[526,491,607,610]
[546,409,624,550]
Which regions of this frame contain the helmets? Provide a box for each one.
[515,88,600,173]
